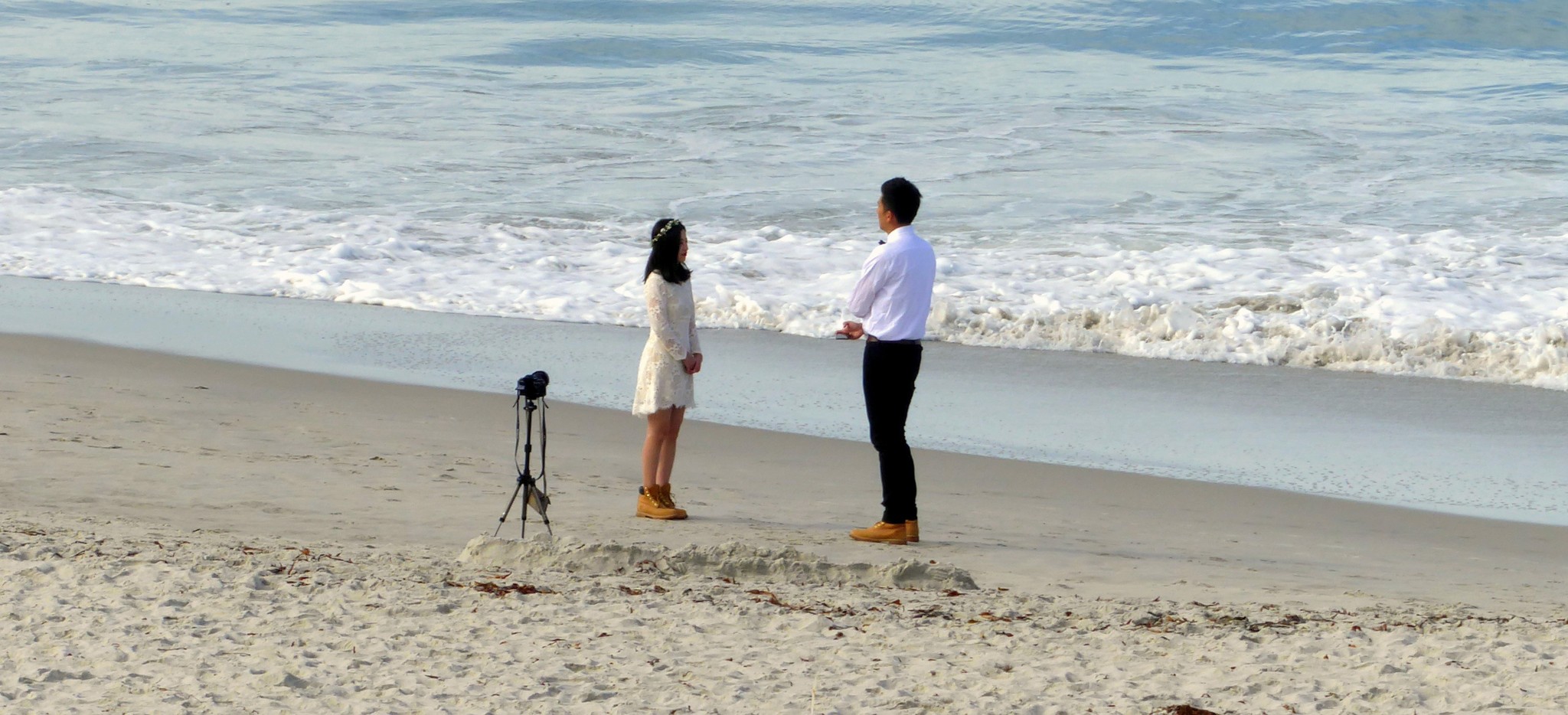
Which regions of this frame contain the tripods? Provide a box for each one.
[494,400,553,540]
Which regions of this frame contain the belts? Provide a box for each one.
[867,334,921,345]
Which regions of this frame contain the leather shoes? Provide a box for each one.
[849,521,907,545]
[905,519,919,542]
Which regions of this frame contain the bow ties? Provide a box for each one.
[878,240,885,244]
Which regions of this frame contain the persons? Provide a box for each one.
[631,219,703,520]
[834,177,937,545]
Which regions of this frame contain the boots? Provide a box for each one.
[658,483,688,519]
[635,484,677,519]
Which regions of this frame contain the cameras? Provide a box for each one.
[515,371,549,400]
